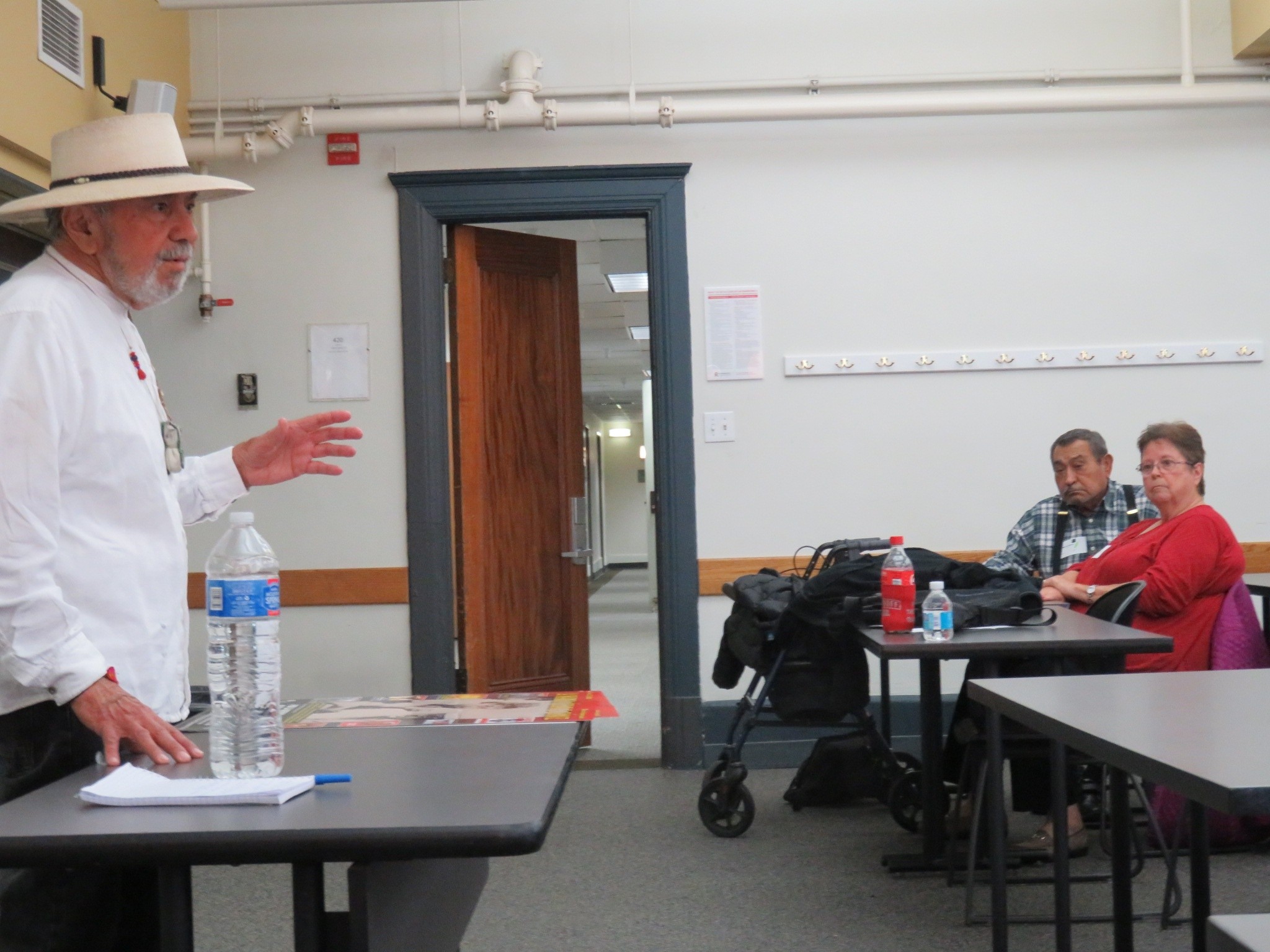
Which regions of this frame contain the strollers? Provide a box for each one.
[696,537,951,839]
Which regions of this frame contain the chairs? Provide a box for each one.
[967,577,1270,923]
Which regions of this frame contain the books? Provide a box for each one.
[79,761,316,806]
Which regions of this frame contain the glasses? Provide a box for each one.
[1136,459,1196,475]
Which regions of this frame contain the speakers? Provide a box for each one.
[124,78,177,117]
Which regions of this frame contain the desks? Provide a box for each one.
[0,691,590,952]
[966,668,1270,952]
[855,611,1174,873]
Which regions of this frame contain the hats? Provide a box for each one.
[0,114,253,223]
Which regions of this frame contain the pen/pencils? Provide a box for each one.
[315,774,353,783]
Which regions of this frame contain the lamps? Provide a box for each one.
[93,37,177,117]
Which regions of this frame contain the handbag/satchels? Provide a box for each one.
[795,547,1057,629]
[783,729,889,808]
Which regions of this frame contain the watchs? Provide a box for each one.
[1086,583,1097,606]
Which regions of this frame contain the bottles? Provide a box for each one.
[880,535,916,634]
[203,511,285,780]
[921,581,953,642]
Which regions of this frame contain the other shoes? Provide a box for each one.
[1012,827,1089,862]
[947,802,974,835]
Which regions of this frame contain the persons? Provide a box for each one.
[941,427,1161,816]
[0,112,364,951]
[1018,423,1248,858]
